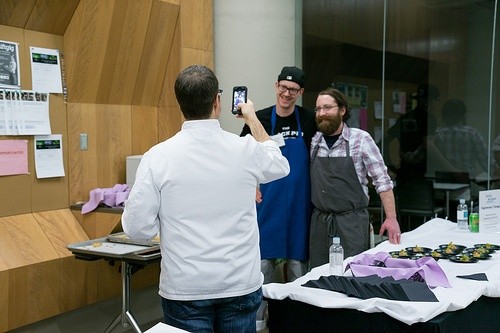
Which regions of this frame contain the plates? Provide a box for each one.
[389,243,500,264]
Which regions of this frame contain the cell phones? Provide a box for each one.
[232,86,248,114]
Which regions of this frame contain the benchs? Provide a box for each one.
[367,205,441,223]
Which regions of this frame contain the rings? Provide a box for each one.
[397,233,400,235]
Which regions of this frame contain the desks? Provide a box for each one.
[262,218,500,333]
[66,232,162,333]
[434,183,468,219]
[69,203,124,240]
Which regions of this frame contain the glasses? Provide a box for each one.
[278,81,302,95]
[314,104,339,112]
[218,88,224,94]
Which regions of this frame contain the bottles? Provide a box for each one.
[457,199,468,231]
[329,236,344,277]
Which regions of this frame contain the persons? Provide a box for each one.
[309,89,402,270]
[120,64,290,333]
[236,95,243,109]
[239,64,318,333]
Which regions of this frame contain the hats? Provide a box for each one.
[278,66,307,88]
